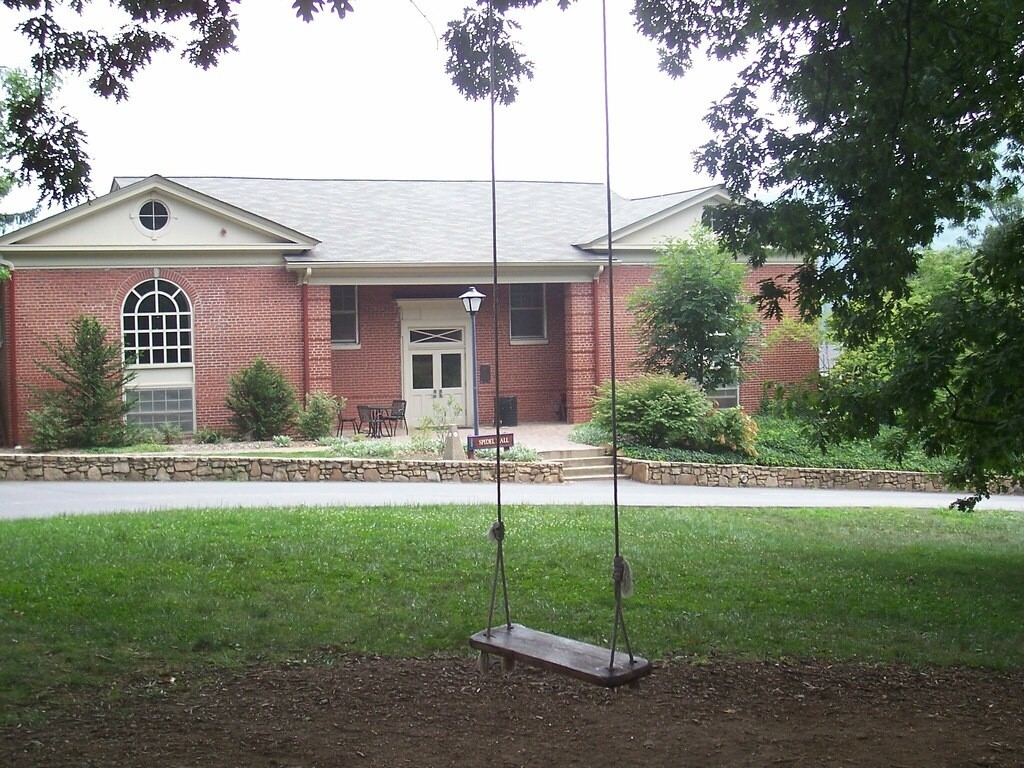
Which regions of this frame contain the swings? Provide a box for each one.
[464,0,658,698]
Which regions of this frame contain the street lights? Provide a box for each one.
[459,287,487,436]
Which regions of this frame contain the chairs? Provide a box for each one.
[357,406,392,438]
[336,403,359,436]
[381,400,408,437]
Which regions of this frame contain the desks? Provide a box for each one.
[366,405,398,438]
